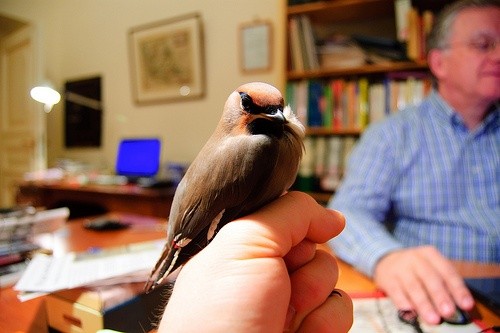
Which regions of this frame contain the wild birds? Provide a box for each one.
[144,81,306,294]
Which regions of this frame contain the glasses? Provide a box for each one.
[447,39,499,53]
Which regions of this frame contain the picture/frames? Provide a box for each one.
[237,20,274,75]
[125,11,208,107]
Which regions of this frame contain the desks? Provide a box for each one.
[313,242,500,333]
[16,181,177,214]
[0,211,168,333]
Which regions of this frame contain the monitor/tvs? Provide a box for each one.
[115,138,161,176]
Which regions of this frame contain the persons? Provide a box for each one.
[158,190,354,333]
[326,0,500,326]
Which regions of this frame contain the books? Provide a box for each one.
[0,206,72,286]
[284,8,443,193]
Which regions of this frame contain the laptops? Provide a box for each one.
[464,278,500,318]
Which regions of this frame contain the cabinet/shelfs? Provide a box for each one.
[280,0,441,201]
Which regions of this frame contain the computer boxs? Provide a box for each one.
[443,307,469,324]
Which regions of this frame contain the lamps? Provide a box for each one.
[23,77,64,182]
